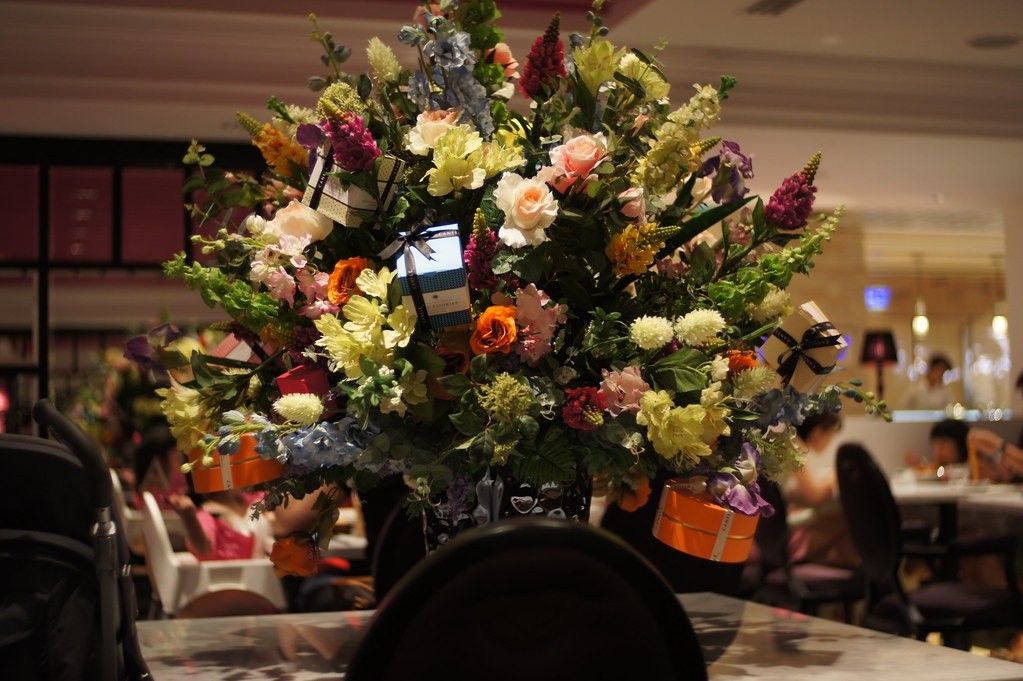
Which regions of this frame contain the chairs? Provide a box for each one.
[1,392,1021,681]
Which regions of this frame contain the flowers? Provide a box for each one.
[120,0,894,521]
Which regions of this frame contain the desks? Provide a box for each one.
[137,592,1022,681]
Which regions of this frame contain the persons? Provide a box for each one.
[908,355,1023,653]
[130,420,177,509]
[783,398,858,564]
[167,456,411,611]
